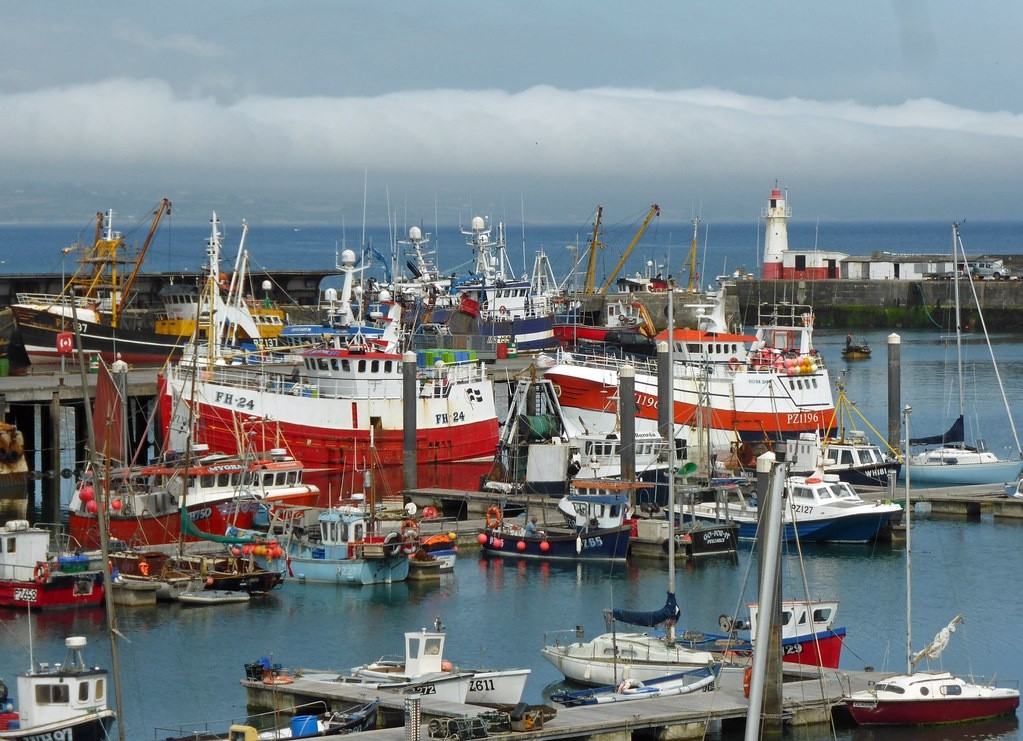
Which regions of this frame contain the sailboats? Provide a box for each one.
[838,403,1023,733]
[545,294,720,692]
[0,294,144,741]
[896,217,1022,488]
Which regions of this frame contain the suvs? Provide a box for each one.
[963,260,1012,281]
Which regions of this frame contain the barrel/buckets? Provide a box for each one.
[290,715,318,737]
[90,356,99,374]
[258,657,269,669]
[8,721,20,729]
[497,342,517,359]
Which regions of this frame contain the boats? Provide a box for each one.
[154,691,381,741]
[841,338,871,358]
[0,197,903,618]
[352,614,537,714]
[653,599,847,672]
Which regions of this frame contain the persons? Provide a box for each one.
[526,517,546,538]
[845,332,851,347]
[525,294,535,317]
[290,362,300,384]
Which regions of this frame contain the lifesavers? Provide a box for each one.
[618,678,645,694]
[263,676,293,684]
[486,506,500,528]
[500,305,506,314]
[384,532,401,556]
[34,563,49,584]
[744,668,752,699]
[728,357,739,372]
[805,478,821,483]
[402,520,418,538]
[401,531,419,554]
